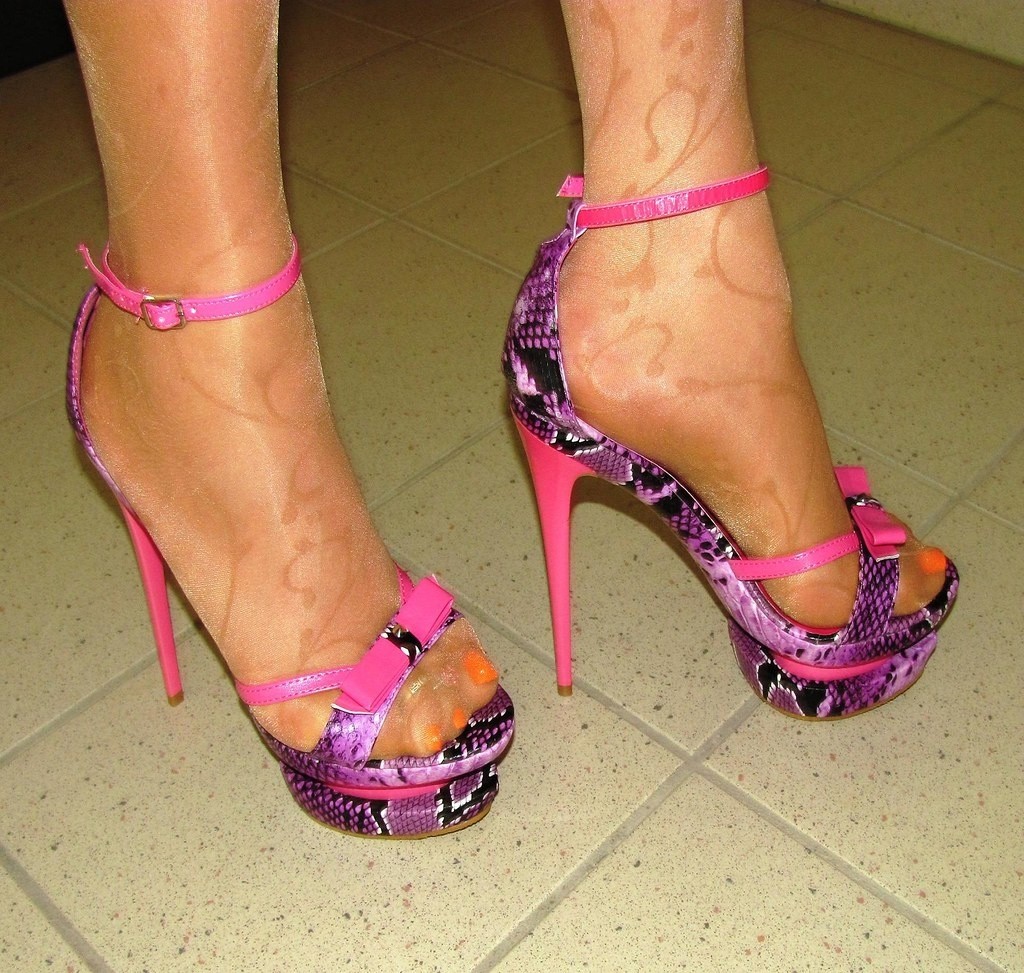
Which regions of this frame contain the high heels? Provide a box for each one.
[66,231,516,840]
[503,166,959,722]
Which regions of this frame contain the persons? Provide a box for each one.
[64,0,959,839]
